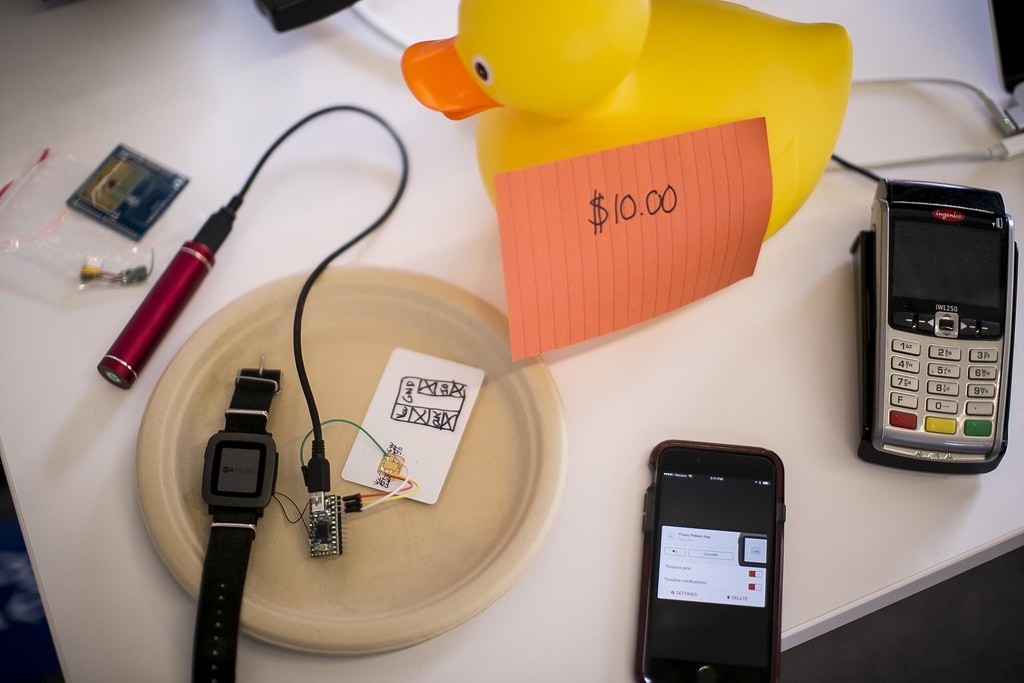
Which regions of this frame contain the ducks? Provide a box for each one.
[401,0,852,244]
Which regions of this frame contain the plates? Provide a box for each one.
[137,266,563,655]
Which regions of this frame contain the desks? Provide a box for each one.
[0,0,1024,683]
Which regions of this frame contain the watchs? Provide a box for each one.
[188,353,284,683]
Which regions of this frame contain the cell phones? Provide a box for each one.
[643,439,785,683]
